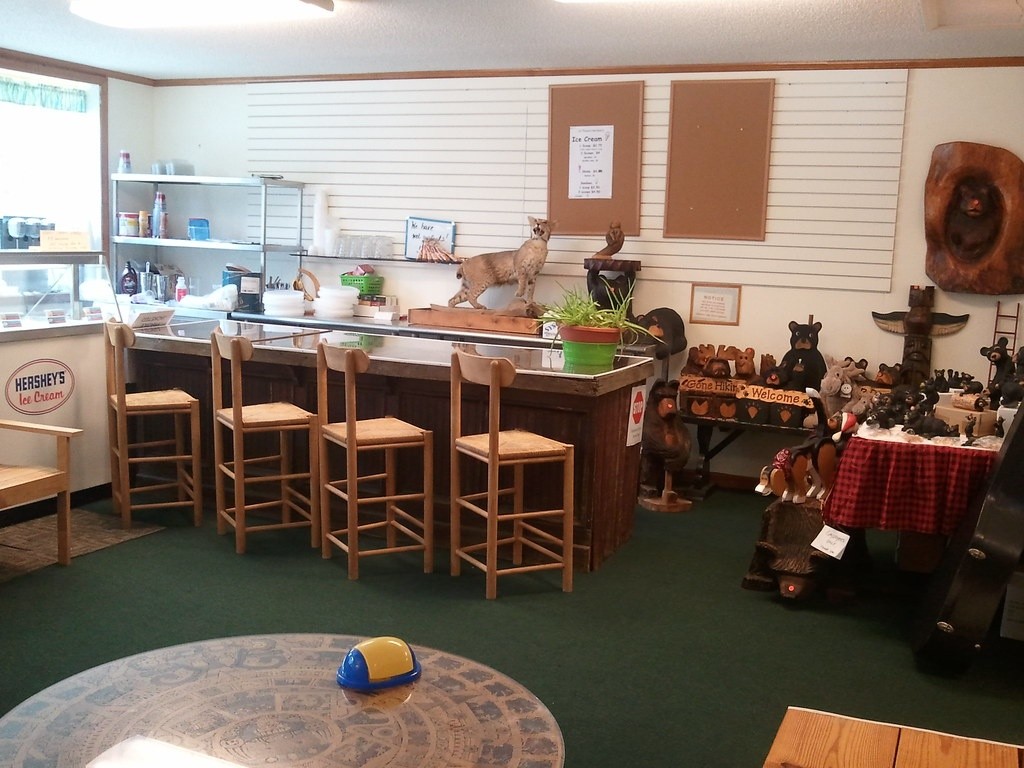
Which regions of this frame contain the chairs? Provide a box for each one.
[0,420,83,565]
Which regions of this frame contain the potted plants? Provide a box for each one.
[529,280,665,361]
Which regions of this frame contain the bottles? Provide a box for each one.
[139,211,148,237]
[175,277,187,302]
[120,262,137,294]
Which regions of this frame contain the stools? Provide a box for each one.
[317,337,434,581]
[450,345,574,600]
[211,325,321,559]
[104,317,201,531]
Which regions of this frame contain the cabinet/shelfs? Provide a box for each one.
[111,171,304,315]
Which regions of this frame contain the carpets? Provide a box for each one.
[0,508,165,583]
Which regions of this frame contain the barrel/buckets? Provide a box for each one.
[118,212,140,237]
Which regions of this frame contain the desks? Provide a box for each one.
[1,632,565,768]
[685,388,1000,593]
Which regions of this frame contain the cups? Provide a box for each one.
[307,189,393,258]
[138,272,169,303]
[188,277,201,296]
[117,150,132,174]
[152,192,167,236]
